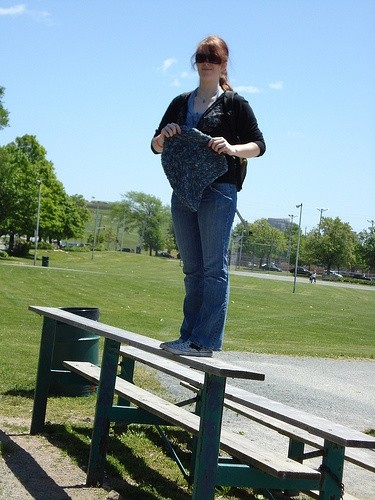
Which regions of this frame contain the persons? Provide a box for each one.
[59,240,62,249]
[150,35,268,357]
[309,272,316,284]
[362,272,365,279]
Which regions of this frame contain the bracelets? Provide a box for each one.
[156,136,162,148]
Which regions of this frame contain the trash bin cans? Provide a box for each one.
[41,255,48,267]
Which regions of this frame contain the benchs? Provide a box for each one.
[27,305,375,500]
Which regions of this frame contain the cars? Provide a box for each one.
[322,270,343,278]
[289,267,313,276]
[262,264,281,272]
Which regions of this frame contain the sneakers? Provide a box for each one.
[159,337,188,349]
[166,339,214,357]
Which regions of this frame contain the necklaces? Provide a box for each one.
[198,85,221,103]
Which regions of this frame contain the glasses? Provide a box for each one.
[196,53,223,64]
